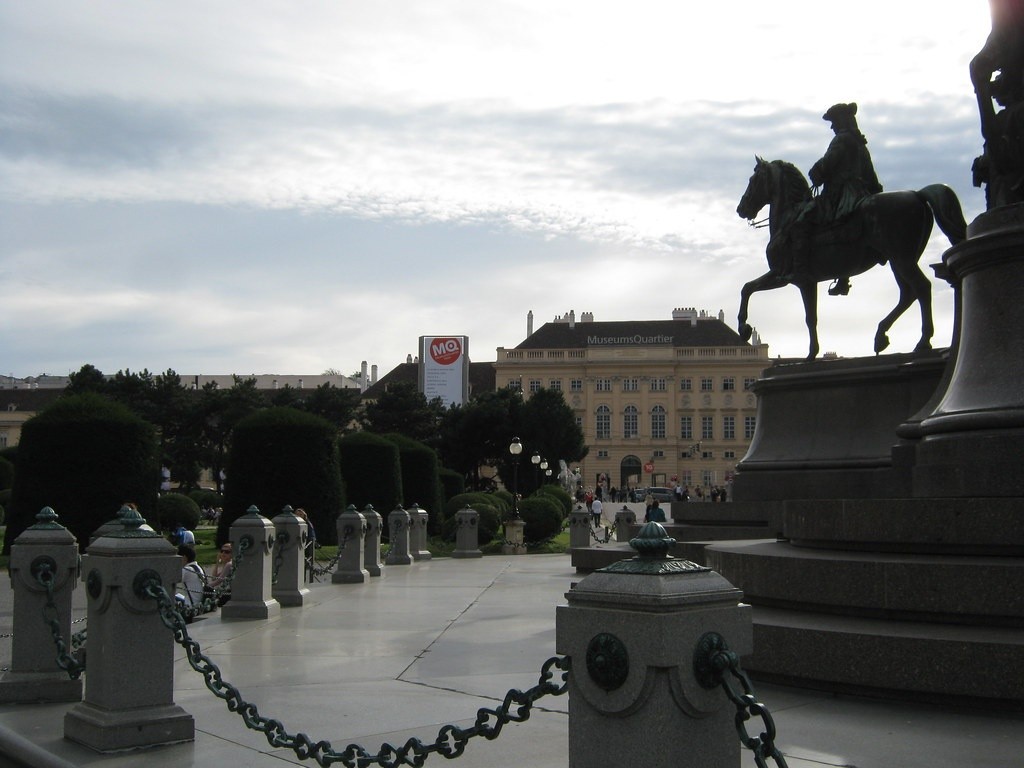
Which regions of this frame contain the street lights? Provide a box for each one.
[531,451,553,491]
[501,438,527,555]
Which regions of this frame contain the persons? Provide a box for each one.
[178,544,206,607]
[645,492,653,506]
[710,485,727,501]
[296,509,314,582]
[199,505,223,524]
[577,484,666,522]
[809,102,882,295]
[592,497,603,528]
[176,522,194,544]
[695,485,705,501]
[675,484,689,501]
[208,543,233,607]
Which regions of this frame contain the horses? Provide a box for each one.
[736,155,969,360]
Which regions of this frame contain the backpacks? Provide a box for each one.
[183,563,216,613]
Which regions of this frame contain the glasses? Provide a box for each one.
[220,549,231,555]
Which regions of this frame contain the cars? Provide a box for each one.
[634,487,674,502]
